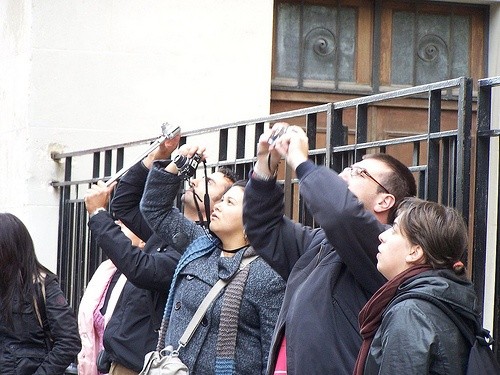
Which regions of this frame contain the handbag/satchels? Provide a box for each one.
[138,346,189,375]
[96,351,112,374]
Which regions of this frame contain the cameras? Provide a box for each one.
[161,122,181,140]
[267,124,288,145]
[174,153,201,177]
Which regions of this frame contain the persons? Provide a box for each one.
[0,212,83,375]
[136,140,287,375]
[83,129,240,374]
[352,200,483,375]
[74,219,146,375]
[240,121,418,374]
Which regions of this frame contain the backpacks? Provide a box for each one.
[383,292,500,375]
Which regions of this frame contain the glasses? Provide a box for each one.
[344,167,390,193]
